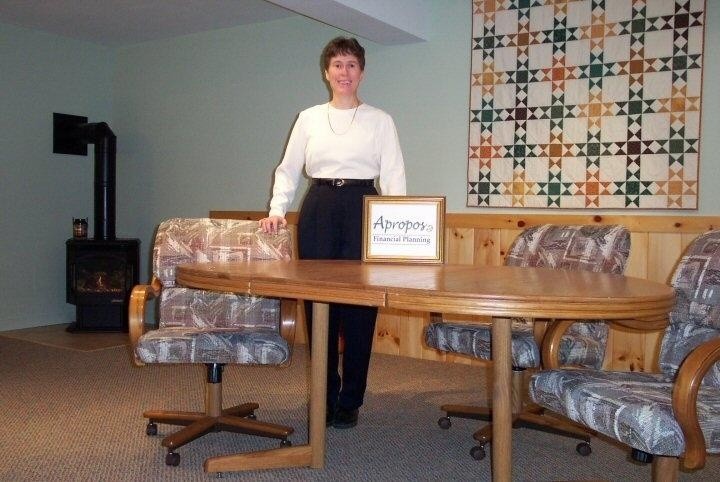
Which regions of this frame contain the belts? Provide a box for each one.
[311,178,374,189]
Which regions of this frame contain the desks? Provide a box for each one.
[176,258,675,482]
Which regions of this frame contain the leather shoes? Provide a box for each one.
[326,405,359,429]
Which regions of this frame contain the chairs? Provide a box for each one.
[529,230,720,482]
[424,223,631,461]
[129,218,298,467]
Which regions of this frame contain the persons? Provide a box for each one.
[258,36,406,428]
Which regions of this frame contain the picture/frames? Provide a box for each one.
[361,195,446,263]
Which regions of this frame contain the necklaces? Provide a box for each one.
[328,102,359,135]
